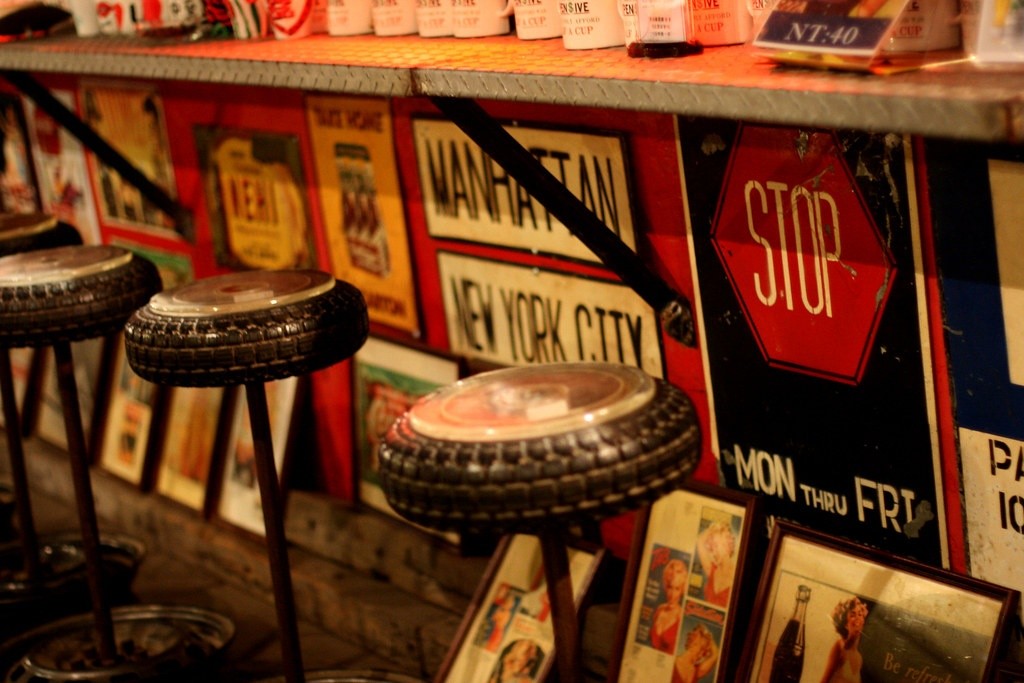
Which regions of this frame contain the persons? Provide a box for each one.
[482,587,517,652]
[697,517,735,608]
[501,643,535,682]
[672,623,717,683]
[820,597,868,683]
[648,559,688,655]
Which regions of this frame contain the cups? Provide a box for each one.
[70,0,1024,62]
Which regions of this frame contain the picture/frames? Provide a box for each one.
[9,332,311,551]
[345,331,469,552]
[433,533,605,683]
[605,479,756,682]
[736,520,1021,683]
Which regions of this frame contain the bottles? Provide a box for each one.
[771,586,811,683]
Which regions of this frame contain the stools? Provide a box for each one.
[125,268,368,683]
[378,362,705,683]
[0,212,148,592]
[0,244,234,683]
